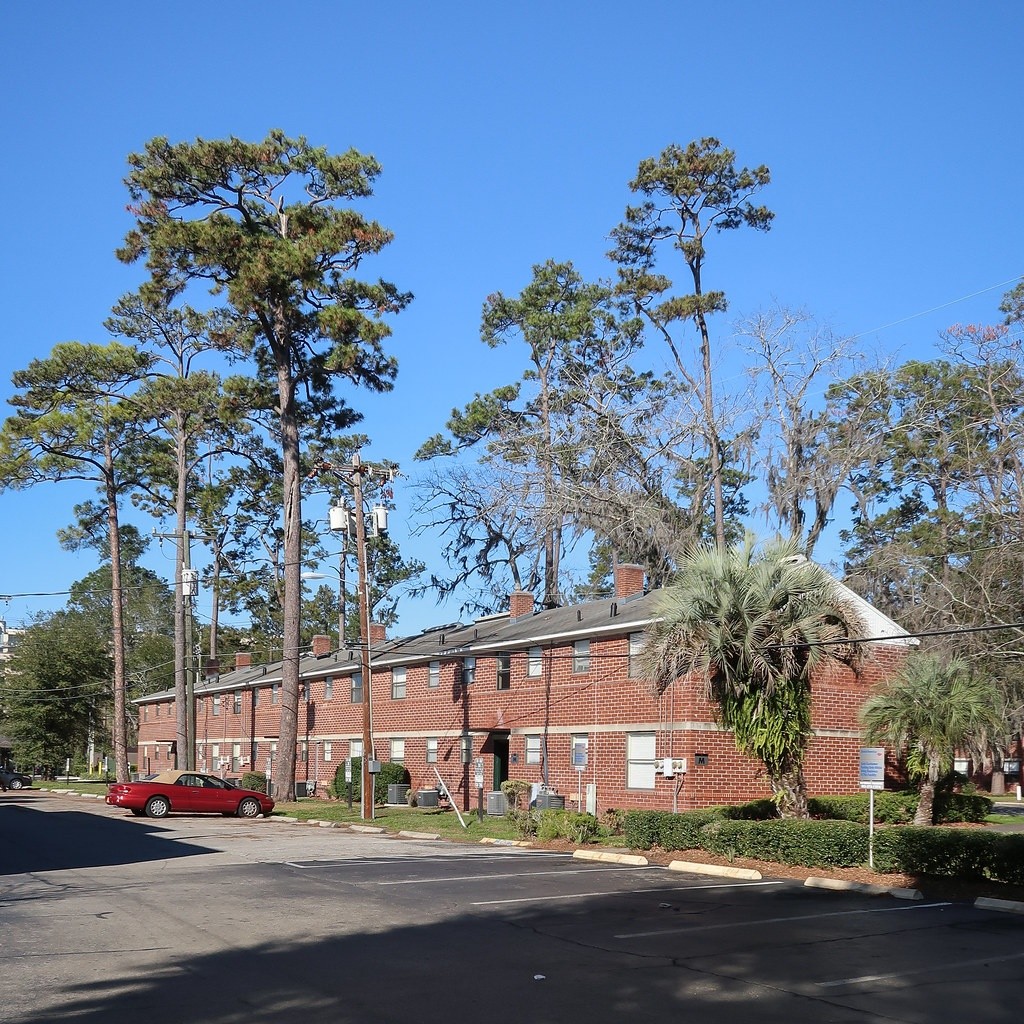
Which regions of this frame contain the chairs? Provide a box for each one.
[175,780,183,786]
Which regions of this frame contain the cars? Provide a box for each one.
[0,768,33,790]
[104,770,275,819]
